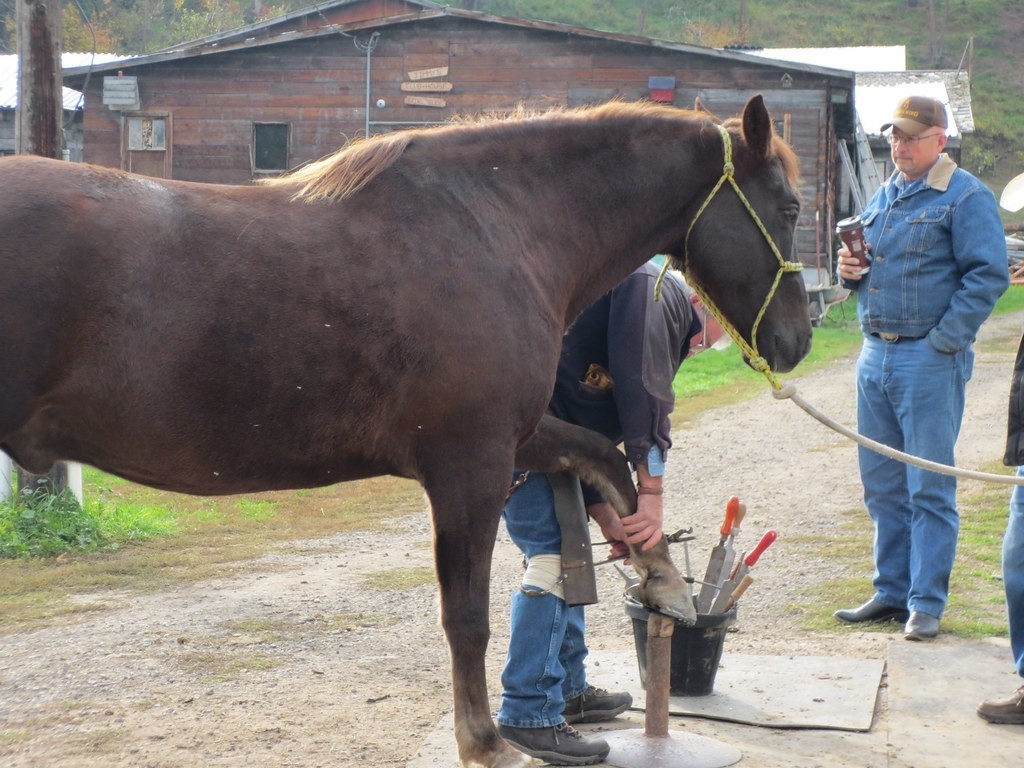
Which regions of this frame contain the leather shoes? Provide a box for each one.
[903,611,939,641]
[977,683,1024,724]
[834,599,908,625]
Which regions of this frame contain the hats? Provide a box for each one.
[880,95,948,135]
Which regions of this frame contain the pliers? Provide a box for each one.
[592,526,696,566]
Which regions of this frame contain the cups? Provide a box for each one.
[836,216,869,274]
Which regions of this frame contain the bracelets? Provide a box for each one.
[637,487,664,495]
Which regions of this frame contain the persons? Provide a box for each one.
[976,169,1024,725]
[490,260,738,765]
[834,94,1011,642]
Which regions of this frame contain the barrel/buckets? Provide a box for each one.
[623,577,736,695]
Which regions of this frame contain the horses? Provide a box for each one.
[2,90,815,767]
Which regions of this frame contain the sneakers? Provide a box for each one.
[560,684,633,725]
[498,722,610,766]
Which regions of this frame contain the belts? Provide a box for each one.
[871,332,925,343]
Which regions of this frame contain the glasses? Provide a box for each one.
[886,133,941,145]
[689,312,707,354]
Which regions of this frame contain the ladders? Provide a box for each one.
[837,138,866,215]
[855,116,882,194]
